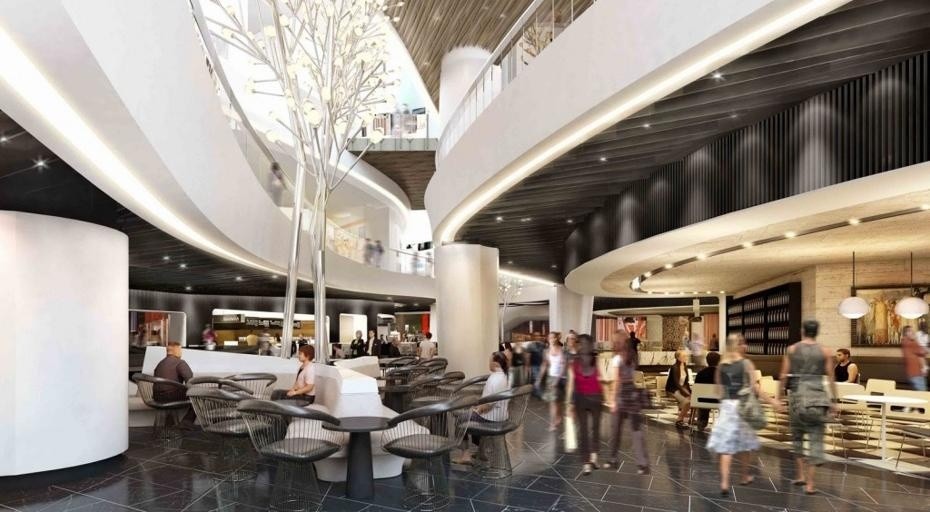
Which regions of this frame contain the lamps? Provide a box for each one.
[838,252,874,320]
[894,251,929,319]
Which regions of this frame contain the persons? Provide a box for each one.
[358,237,375,263]
[246,329,259,346]
[529,329,649,476]
[269,344,317,404]
[350,330,364,358]
[773,321,838,495]
[709,333,719,351]
[333,344,347,359]
[151,340,201,431]
[912,322,930,376]
[395,251,402,273]
[291,333,308,358]
[273,332,280,342]
[418,332,435,360]
[374,239,385,270]
[148,330,160,346]
[832,348,858,383]
[425,252,433,276]
[267,162,290,207]
[135,324,146,347]
[665,350,692,427]
[698,331,781,495]
[379,334,386,345]
[452,351,514,465]
[401,334,408,343]
[695,352,728,430]
[900,325,930,392]
[411,252,419,274]
[201,324,218,351]
[500,337,533,385]
[365,329,382,360]
